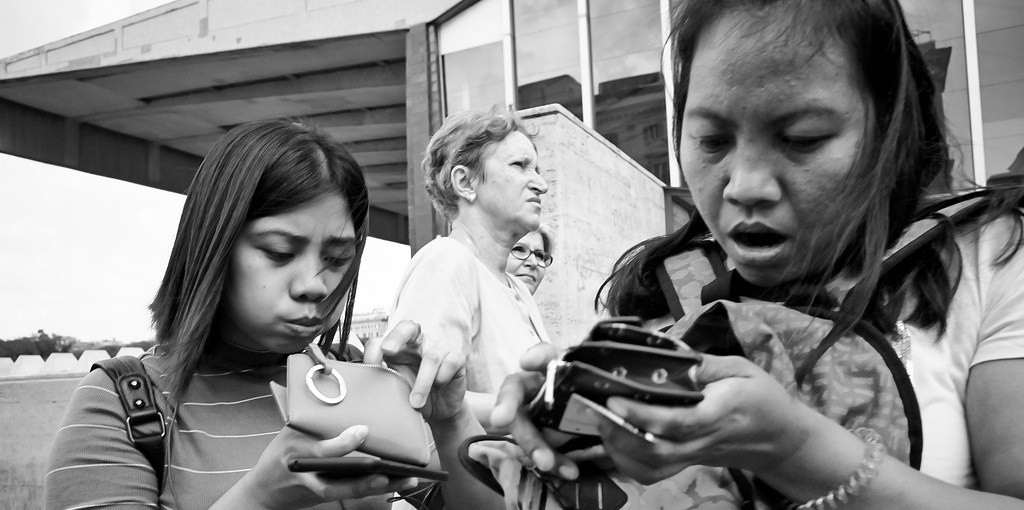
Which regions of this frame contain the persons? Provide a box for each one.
[503,215,553,299]
[379,107,560,438]
[489,0,1024,510]
[45,120,511,509]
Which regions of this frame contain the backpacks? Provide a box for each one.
[456,189,1006,510]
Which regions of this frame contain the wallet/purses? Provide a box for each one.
[269,353,431,468]
[534,315,705,440]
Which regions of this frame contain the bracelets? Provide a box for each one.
[784,425,885,510]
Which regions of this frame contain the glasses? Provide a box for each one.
[510,243,553,268]
